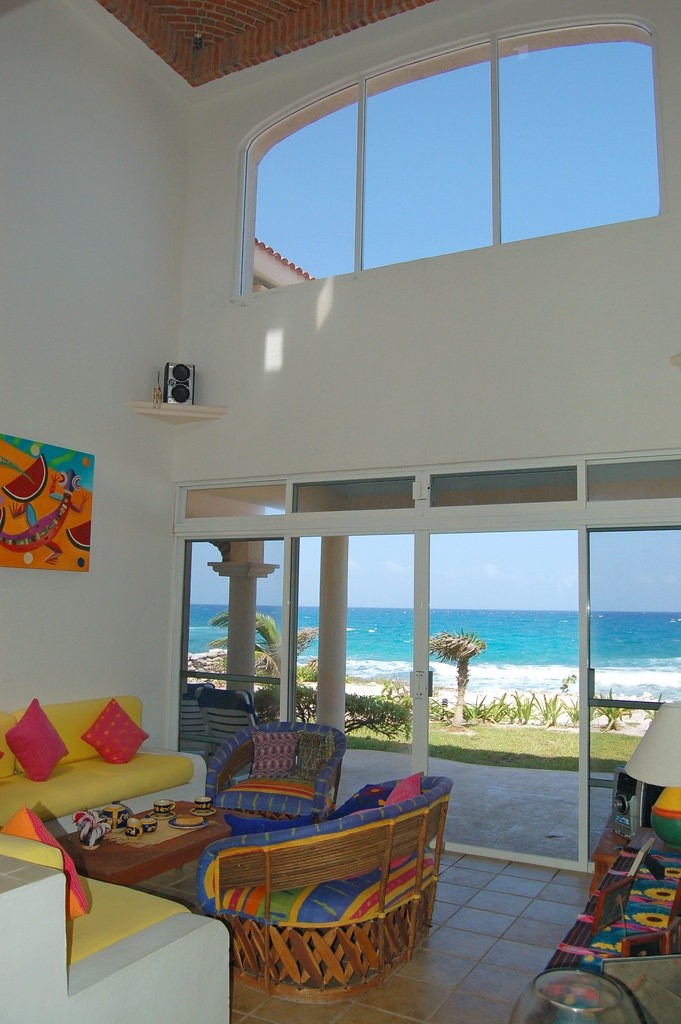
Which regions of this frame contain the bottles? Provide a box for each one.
[509,967,640,1024]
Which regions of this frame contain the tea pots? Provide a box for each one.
[102,801,129,832]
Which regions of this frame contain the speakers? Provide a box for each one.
[163,362,195,405]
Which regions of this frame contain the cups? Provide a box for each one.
[154,799,175,817]
[140,817,157,832]
[194,796,212,813]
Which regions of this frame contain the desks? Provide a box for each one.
[57,802,263,884]
[543,827,681,1024]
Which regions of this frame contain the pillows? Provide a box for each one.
[79,697,149,766]
[332,772,421,883]
[2,804,88,922]
[5,698,70,782]
[247,730,301,780]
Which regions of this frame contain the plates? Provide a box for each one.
[189,808,216,816]
[167,818,208,829]
[147,811,176,820]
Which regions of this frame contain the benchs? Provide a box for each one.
[0,833,232,1024]
[197,773,454,1004]
[0,695,207,840]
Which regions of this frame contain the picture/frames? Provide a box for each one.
[588,837,681,1024]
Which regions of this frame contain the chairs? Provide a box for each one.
[179,681,257,758]
[204,722,346,825]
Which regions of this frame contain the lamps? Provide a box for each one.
[624,703,681,848]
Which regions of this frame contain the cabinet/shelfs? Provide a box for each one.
[589,825,626,898]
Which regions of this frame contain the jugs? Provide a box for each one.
[125,818,143,840]
